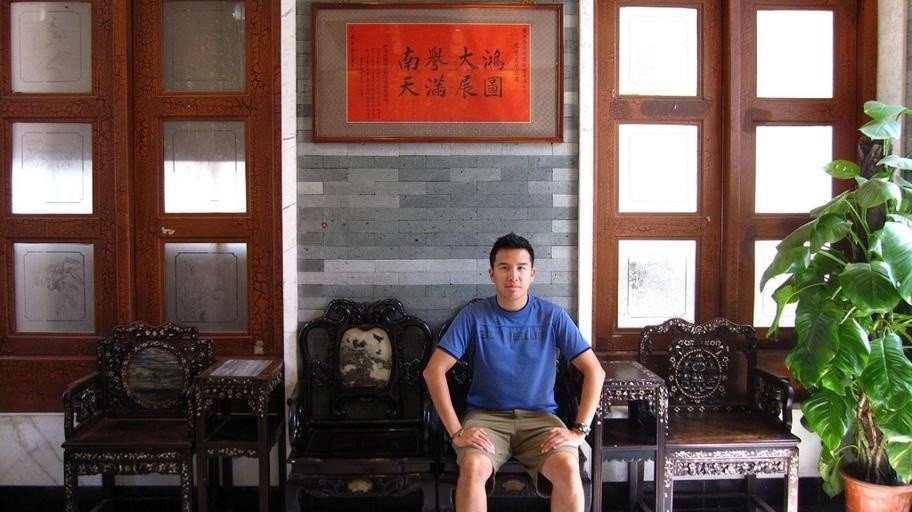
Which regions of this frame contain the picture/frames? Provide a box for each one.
[309,0,565,146]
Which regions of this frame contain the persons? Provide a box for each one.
[422,233,607,512]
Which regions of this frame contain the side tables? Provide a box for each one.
[196,355,285,511]
[568,358,668,511]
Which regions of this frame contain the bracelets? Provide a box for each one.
[573,422,592,435]
[453,429,463,440]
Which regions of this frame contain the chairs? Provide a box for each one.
[637,318,801,511]
[61,321,215,511]
[286,299,437,511]
[430,299,591,511]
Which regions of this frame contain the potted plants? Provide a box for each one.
[758,101,912,512]
